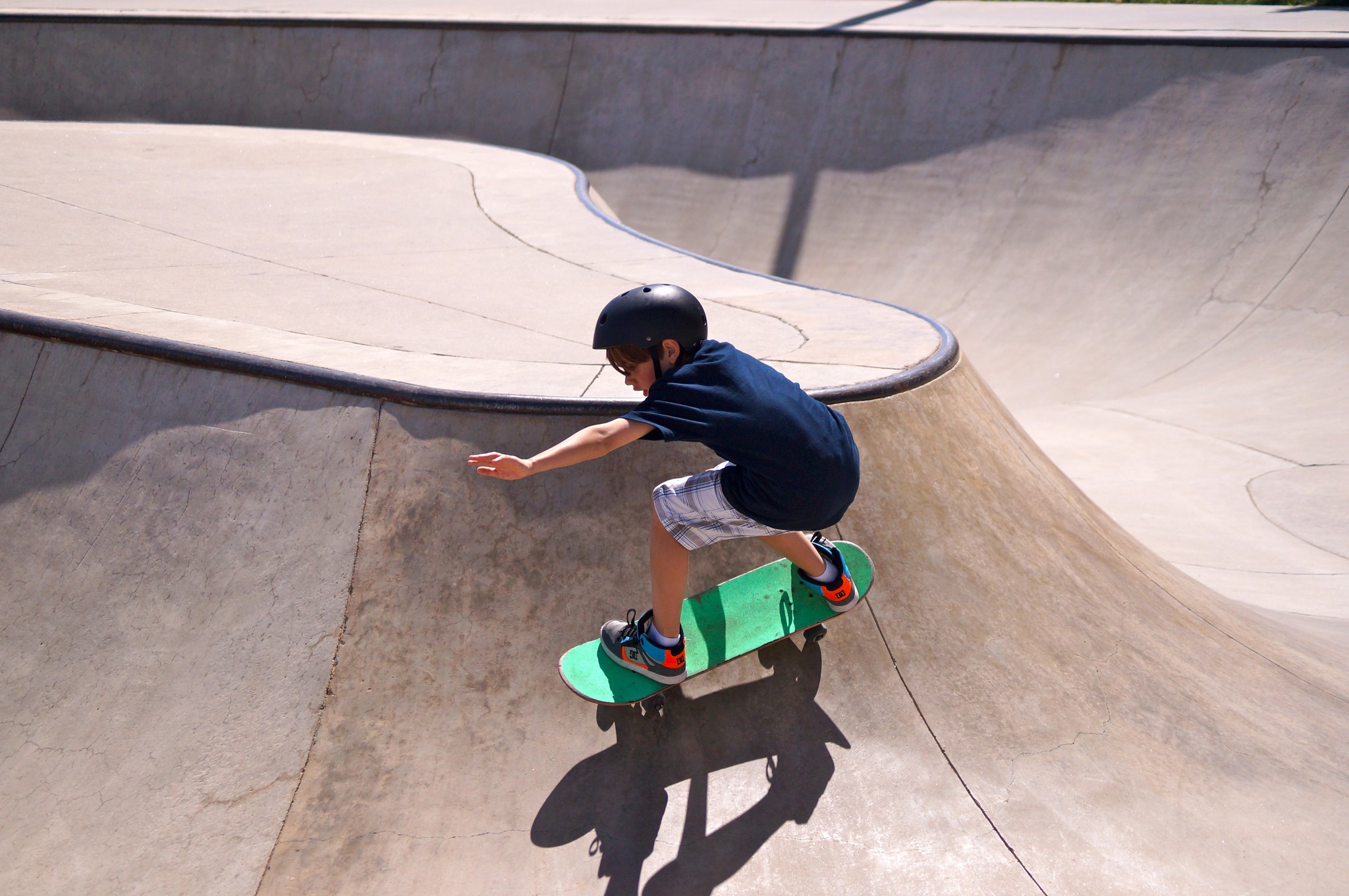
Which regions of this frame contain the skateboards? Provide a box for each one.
[557,541,876,714]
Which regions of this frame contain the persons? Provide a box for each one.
[468,285,861,686]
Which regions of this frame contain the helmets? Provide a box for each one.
[592,283,708,354]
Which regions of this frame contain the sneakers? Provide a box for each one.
[600,608,687,685]
[797,531,859,612]
